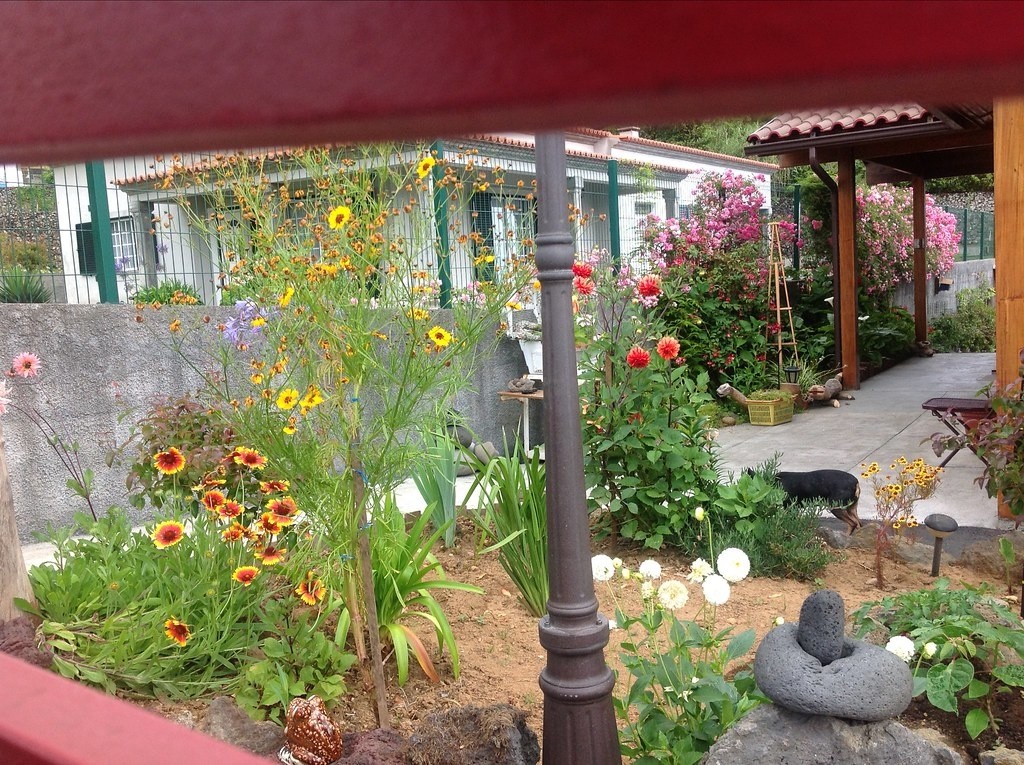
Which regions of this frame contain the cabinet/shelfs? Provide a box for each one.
[762,221,799,391]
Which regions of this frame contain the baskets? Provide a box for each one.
[745,389,794,425]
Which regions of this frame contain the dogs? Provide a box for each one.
[746,467,864,538]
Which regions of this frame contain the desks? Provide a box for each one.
[922,397,999,476]
[495,376,610,462]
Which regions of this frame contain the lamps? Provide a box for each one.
[924,513,959,578]
[768,346,802,390]
